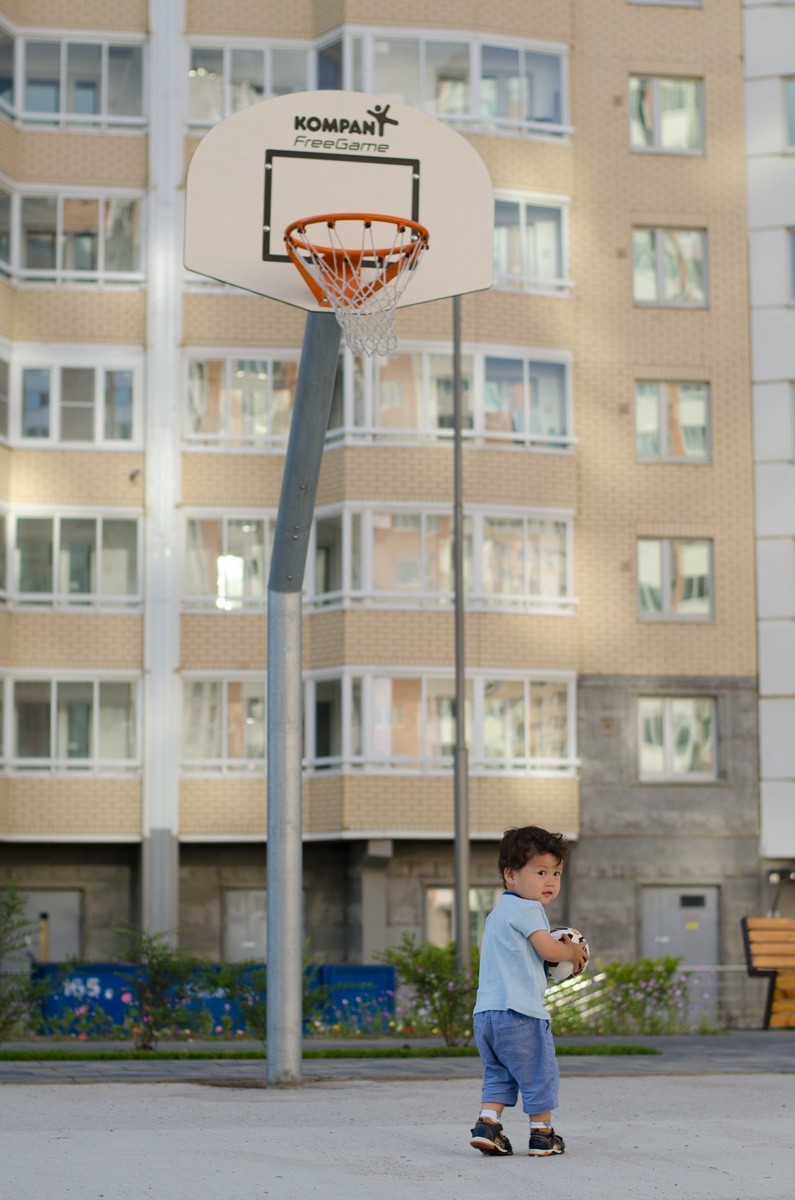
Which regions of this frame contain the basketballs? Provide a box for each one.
[544,924,589,982]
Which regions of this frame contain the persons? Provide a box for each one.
[469,826,588,1156]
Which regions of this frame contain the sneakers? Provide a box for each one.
[470,1120,515,1157]
[529,1129,564,1156]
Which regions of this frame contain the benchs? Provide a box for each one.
[740,916,795,1032]
